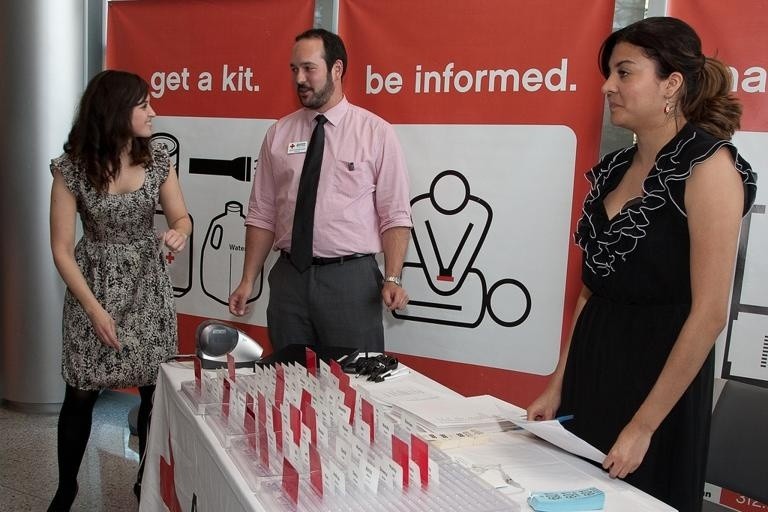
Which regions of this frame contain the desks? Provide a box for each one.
[138,352,680,512]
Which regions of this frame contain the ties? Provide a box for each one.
[290,113,329,274]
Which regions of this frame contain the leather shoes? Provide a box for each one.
[46,480,79,512]
[133,483,141,507]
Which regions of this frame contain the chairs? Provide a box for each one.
[703,378,768,510]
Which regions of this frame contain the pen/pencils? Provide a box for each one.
[555,413,577,423]
[367,368,413,383]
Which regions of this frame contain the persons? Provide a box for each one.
[48,69,194,511]
[524,16,760,512]
[226,28,413,353]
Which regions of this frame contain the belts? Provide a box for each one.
[281,248,372,266]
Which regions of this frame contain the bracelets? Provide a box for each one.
[384,276,402,287]
